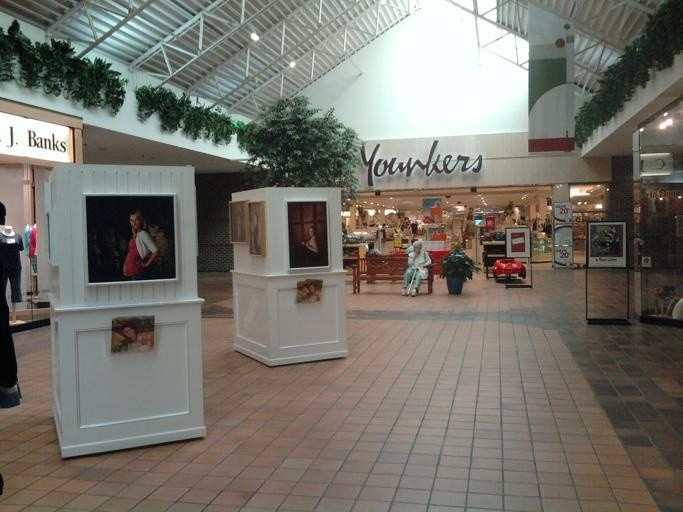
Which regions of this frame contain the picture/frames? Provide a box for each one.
[246,200,267,258]
[505,226,532,258]
[286,200,332,272]
[83,193,179,287]
[228,200,247,246]
[586,220,627,269]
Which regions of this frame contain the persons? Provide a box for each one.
[23,224,30,257]
[28,223,37,297]
[119,209,159,282]
[299,225,320,268]
[400,238,432,297]
[0,199,22,409]
[1,225,24,326]
[113,326,155,352]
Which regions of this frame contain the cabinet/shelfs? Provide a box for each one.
[483,240,515,273]
[342,243,367,274]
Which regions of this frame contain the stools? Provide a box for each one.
[493,258,526,283]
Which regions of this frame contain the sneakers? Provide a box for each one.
[412,288,417,296]
[402,289,406,296]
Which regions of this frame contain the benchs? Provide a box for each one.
[351,254,437,295]
[365,251,436,284]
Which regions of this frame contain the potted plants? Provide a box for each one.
[438,245,482,295]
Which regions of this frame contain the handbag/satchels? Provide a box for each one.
[422,267,429,280]
